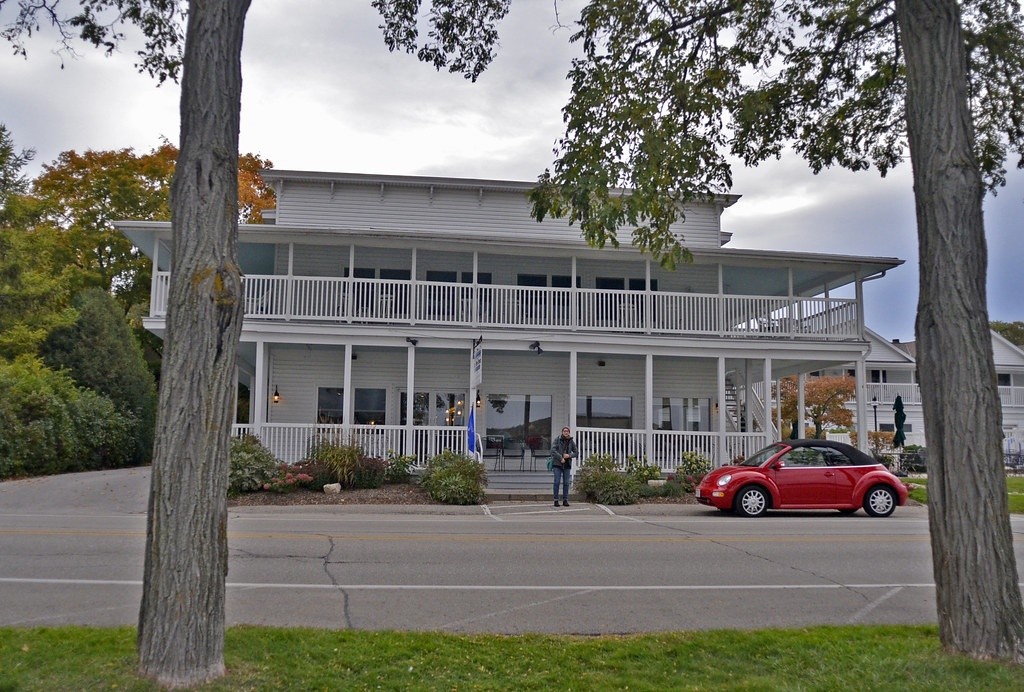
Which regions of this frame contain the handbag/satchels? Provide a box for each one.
[547,457,553,471]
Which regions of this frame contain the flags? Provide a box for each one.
[468,406,474,453]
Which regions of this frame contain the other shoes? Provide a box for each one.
[563,500,569,506]
[554,501,560,506]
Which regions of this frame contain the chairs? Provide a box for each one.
[502,438,525,471]
[528,437,552,472]
[475,437,501,471]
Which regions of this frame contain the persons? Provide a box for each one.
[551,427,578,506]
[741,413,745,432]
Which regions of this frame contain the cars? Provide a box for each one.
[696,438,910,518]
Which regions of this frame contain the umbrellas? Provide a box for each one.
[892,396,906,471]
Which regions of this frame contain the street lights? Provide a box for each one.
[869,396,882,455]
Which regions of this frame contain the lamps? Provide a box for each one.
[407,338,417,345]
[716,404,719,414]
[530,341,544,355]
[273,385,279,403]
